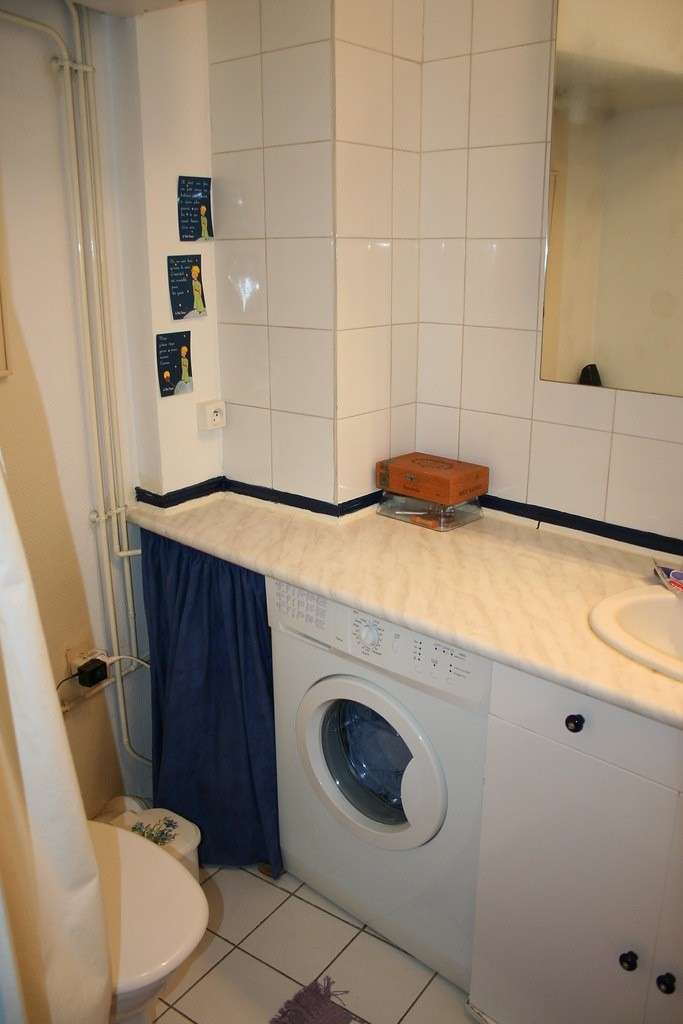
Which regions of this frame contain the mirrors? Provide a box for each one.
[540,0,683,399]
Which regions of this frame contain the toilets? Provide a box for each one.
[84,816,209,1017]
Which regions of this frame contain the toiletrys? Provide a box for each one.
[650,555,683,600]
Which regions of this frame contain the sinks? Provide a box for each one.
[589,580,683,683]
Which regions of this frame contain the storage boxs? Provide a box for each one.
[376,452,489,507]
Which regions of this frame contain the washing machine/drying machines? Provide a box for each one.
[265,573,490,993]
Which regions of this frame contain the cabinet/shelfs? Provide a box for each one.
[469,661,683,1024]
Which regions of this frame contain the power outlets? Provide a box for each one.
[70,648,109,690]
[196,399,226,431]
